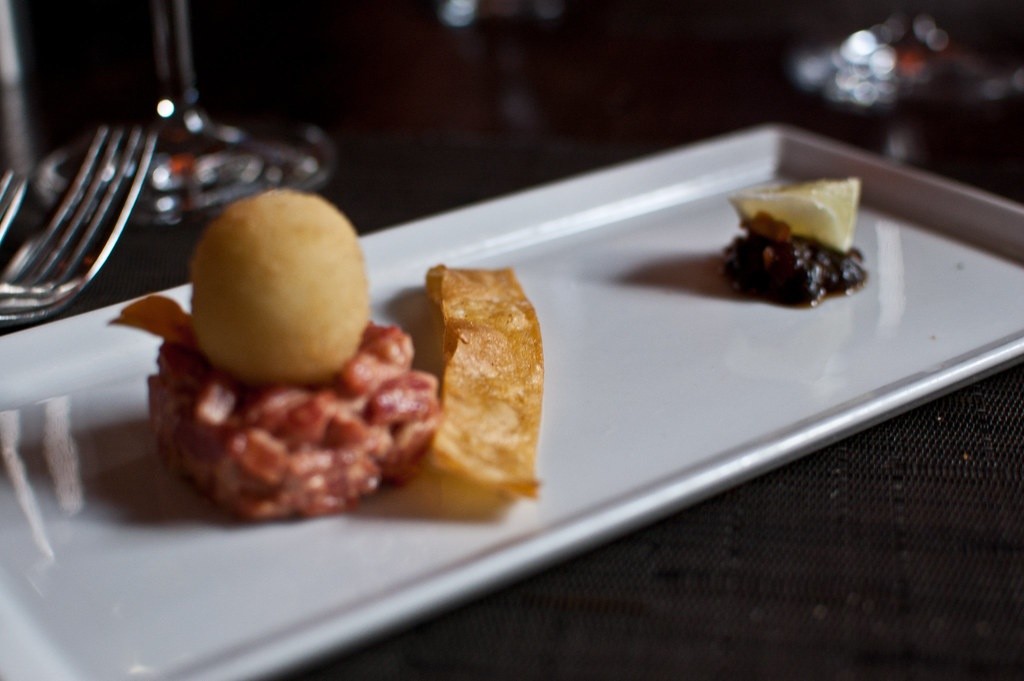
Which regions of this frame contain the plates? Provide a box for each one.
[0,125,1022,681]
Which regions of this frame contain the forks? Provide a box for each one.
[1,127,157,329]
